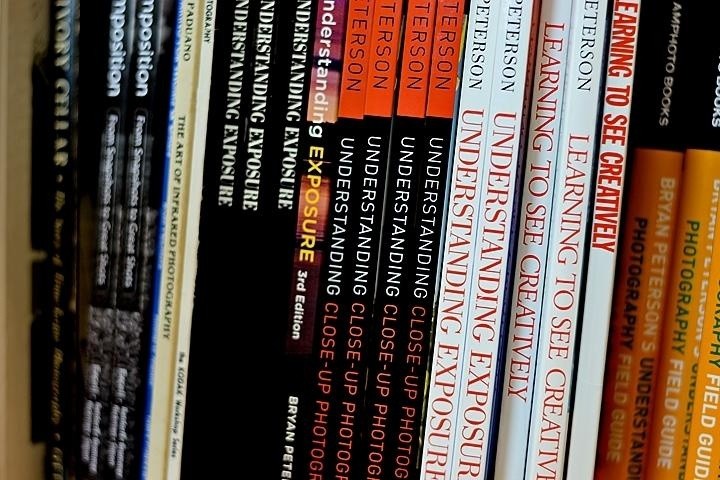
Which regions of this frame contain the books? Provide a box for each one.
[45,0,720,480]
